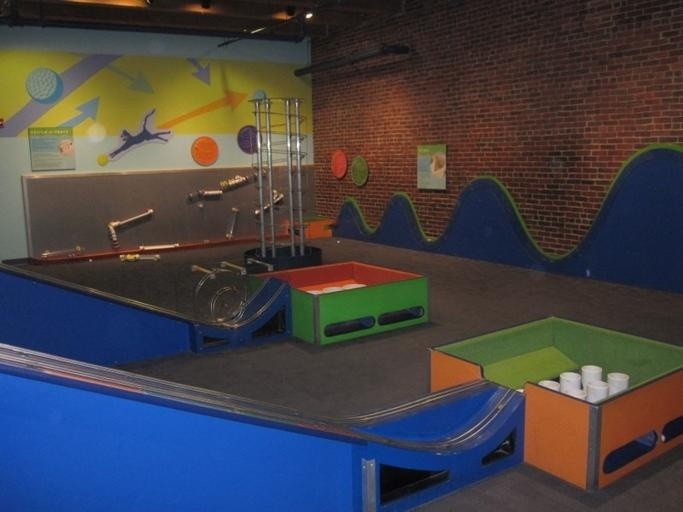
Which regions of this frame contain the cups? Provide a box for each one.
[516,364,631,406]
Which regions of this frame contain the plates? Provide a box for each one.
[304,283,368,296]
